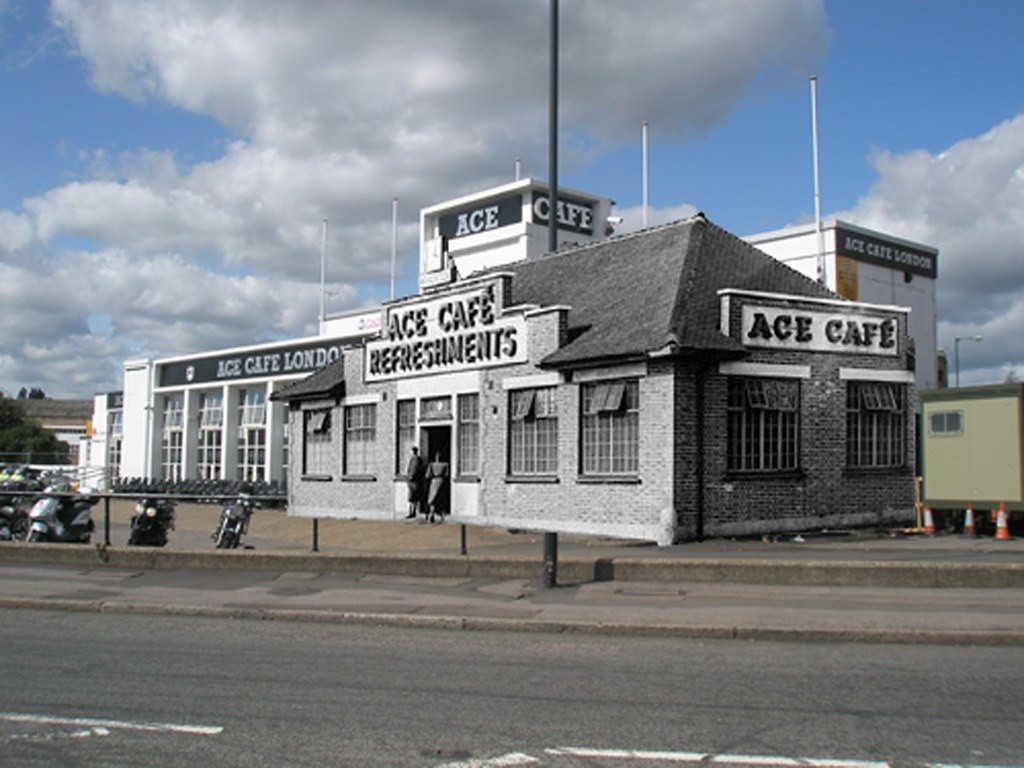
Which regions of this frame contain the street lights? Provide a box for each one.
[955,335,983,388]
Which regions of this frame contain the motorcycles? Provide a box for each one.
[27,479,104,548]
[213,490,260,553]
[0,490,24,545]
[126,499,176,548]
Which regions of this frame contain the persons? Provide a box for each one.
[425,451,449,523]
[406,446,427,518]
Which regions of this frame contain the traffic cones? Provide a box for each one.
[922,503,934,538]
[958,502,979,539]
[990,501,1016,541]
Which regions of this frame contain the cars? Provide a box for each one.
[2,466,72,490]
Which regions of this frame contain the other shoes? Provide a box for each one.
[406,511,417,519]
[426,515,436,525]
